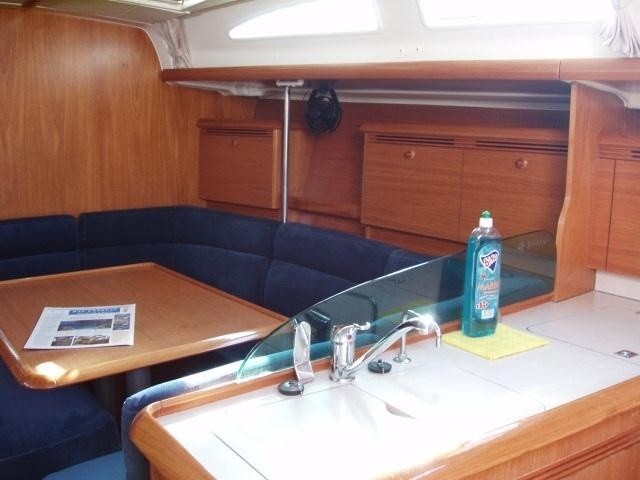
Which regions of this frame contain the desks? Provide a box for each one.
[0,262,294,397]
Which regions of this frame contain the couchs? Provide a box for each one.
[3,205,548,479]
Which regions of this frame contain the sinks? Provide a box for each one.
[214,384,465,480]
[350,365,548,443]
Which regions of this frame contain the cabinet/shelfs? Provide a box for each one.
[360,132,566,254]
[199,128,283,209]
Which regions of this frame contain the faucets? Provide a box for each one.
[330,313,432,382]
